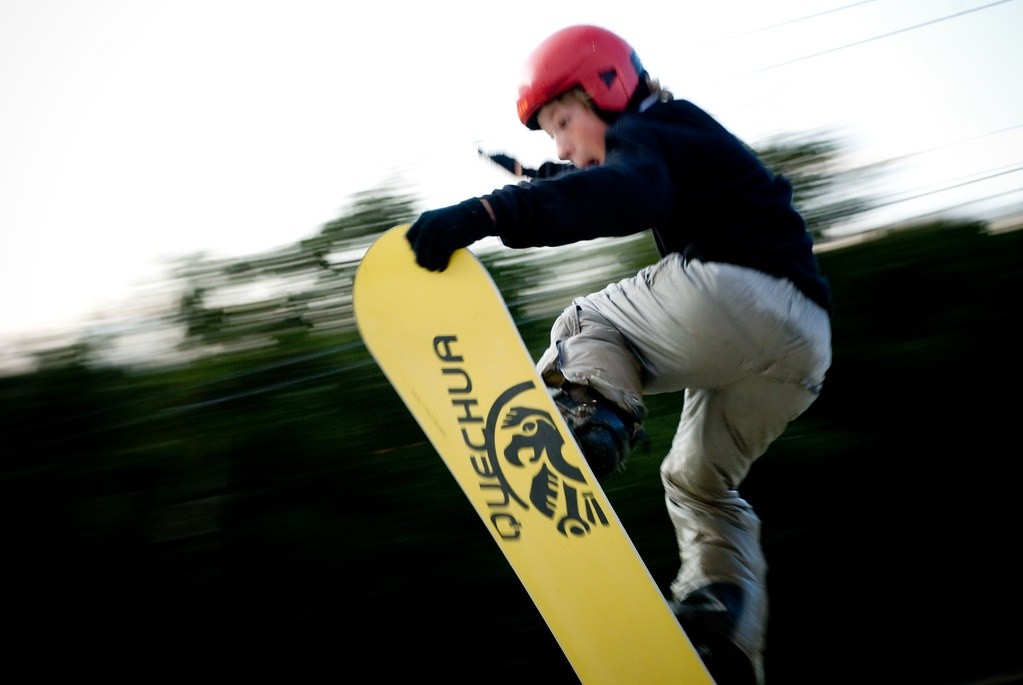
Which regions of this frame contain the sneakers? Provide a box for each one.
[553,384,647,480]
[676,611,766,685]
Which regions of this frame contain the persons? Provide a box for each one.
[406,25,834,685]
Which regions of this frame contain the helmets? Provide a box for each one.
[516,24,652,131]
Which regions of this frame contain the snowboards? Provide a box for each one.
[349,221,717,685]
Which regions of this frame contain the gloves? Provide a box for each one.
[405,197,500,273]
